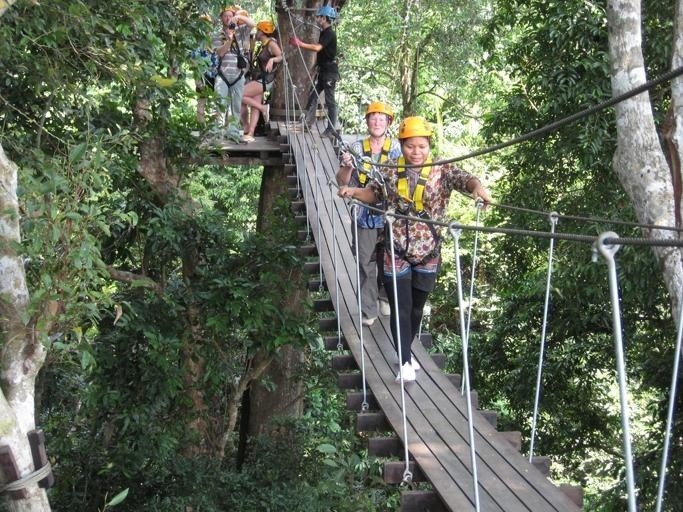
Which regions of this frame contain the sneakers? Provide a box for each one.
[394,353,421,384]
[362,296,391,327]
[293,121,334,138]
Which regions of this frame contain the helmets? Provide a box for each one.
[366,102,394,126]
[198,5,248,25]
[397,116,432,140]
[257,20,274,34]
[315,6,337,19]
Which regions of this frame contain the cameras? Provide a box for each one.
[229,20,236,30]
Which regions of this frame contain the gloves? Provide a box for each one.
[289,36,302,48]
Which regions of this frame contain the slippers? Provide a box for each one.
[262,104,270,123]
[243,134,254,142]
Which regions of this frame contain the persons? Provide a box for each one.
[189,4,283,141]
[336,102,403,325]
[290,6,339,139]
[338,115,490,383]
